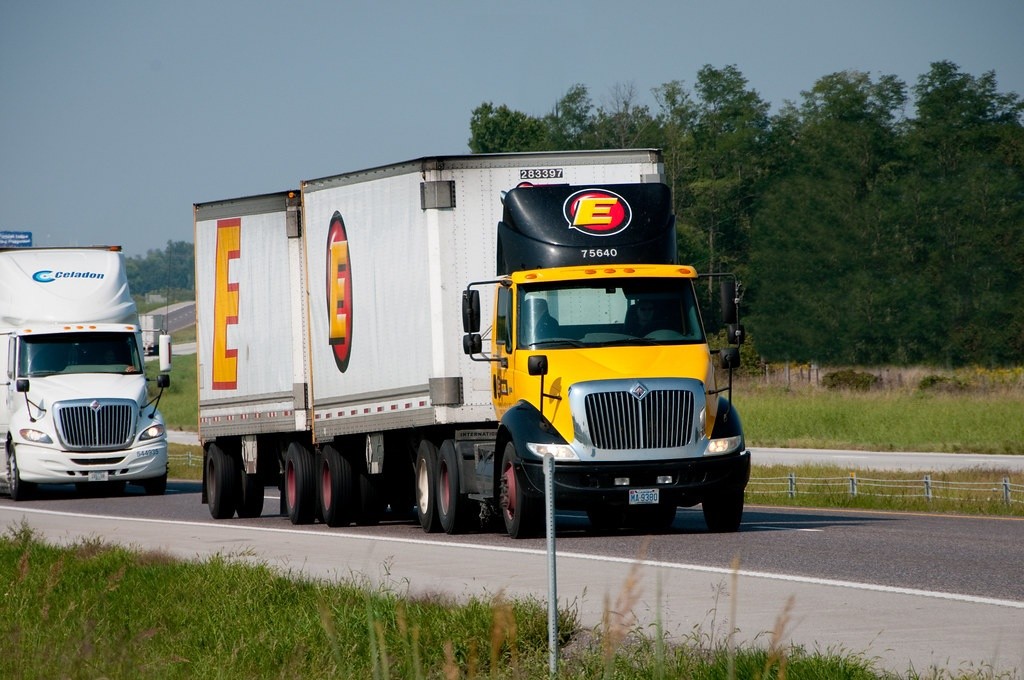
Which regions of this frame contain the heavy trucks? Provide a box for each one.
[190,147,755,541]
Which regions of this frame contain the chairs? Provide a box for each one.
[522,299,559,340]
[623,304,638,336]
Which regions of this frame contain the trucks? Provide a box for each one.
[138,315,164,355]
[0,243,176,500]
